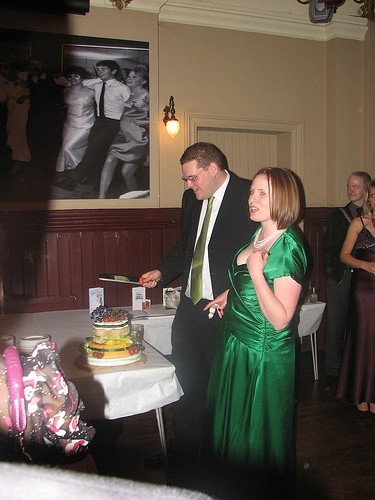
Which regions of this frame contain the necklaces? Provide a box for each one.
[254,226,279,249]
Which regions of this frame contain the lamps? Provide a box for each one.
[163,96,180,137]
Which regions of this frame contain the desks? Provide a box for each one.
[0,299,327,464]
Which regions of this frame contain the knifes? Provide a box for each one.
[97,273,158,288]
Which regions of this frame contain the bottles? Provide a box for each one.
[308,284,312,297]
[310,287,317,303]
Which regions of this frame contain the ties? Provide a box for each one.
[190,196,216,305]
[99,83,106,119]
[356,208,363,217]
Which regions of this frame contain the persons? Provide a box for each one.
[201,167,312,500]
[322,171,372,390]
[0,54,149,199]
[139,142,261,497]
[336,180,375,416]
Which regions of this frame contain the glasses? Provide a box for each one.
[181,163,209,183]
[369,194,375,198]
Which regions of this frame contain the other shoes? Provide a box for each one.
[368,402,375,420]
[47,169,76,191]
[352,402,370,420]
[323,384,335,391]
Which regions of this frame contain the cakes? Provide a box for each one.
[79,304,139,358]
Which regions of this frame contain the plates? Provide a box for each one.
[0,335,14,351]
[20,334,51,354]
